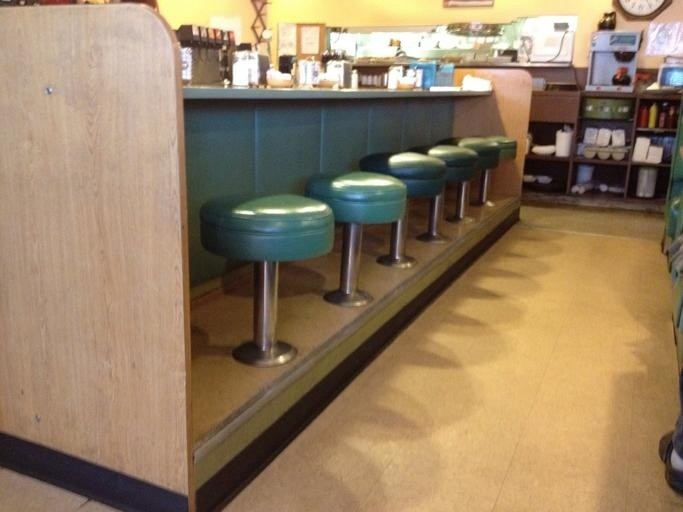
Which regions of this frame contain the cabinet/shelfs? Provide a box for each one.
[523,94,682,206]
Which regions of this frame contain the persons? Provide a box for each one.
[658,411,683,495]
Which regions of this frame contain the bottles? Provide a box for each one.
[638,102,676,129]
[232,51,258,89]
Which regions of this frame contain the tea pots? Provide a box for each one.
[599,12,616,31]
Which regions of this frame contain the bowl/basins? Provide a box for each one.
[397,84,415,90]
[317,80,335,88]
[268,80,294,88]
[532,146,555,155]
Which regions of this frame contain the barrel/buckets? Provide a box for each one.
[637,168,657,198]
[555,130,572,157]
[577,166,594,185]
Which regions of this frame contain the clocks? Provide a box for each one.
[611,0,673,22]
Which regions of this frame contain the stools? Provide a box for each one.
[450,135,498,229]
[416,141,478,244]
[201,189,335,368]
[303,170,407,309]
[470,131,517,211]
[364,149,450,270]
